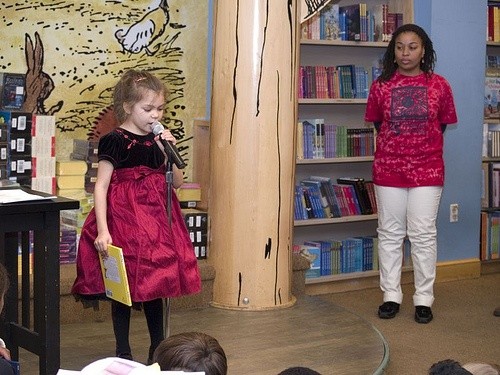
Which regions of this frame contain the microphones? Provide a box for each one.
[152,121,186,170]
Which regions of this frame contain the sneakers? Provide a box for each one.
[378,300,399,318]
[416,306,433,324]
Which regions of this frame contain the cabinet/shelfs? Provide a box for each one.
[479,0,500,281]
[289,1,404,293]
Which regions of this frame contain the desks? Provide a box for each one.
[0,185,81,375]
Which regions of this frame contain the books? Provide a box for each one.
[478,1,500,260]
[98,242,133,308]
[296,0,416,278]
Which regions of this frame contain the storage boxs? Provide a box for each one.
[0,72,209,263]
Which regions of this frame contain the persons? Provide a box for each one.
[362,23,457,323]
[429,358,473,375]
[151,331,230,375]
[69,68,202,361]
[0,264,19,375]
